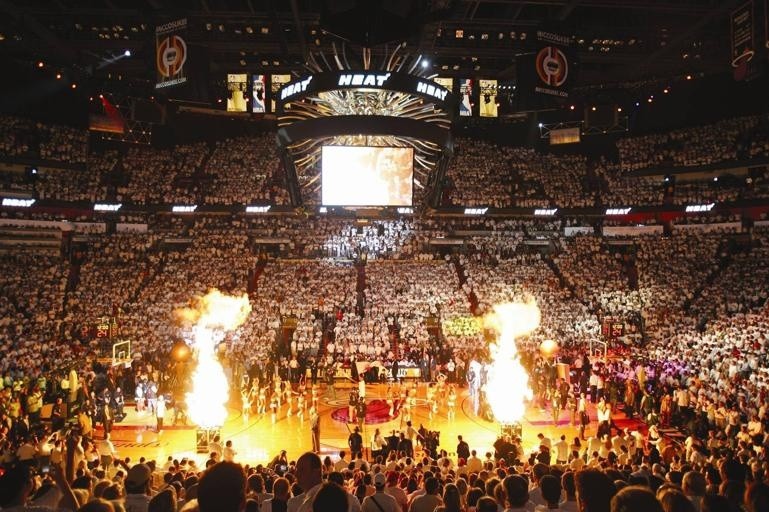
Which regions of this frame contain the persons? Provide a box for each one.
[460,79,472,115]
[253,75,266,113]
[0,112,768,511]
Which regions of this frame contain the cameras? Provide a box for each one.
[40,464,55,474]
